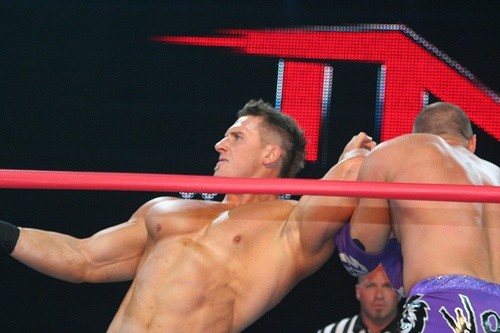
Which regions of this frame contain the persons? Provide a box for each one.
[337,102,500,333]
[0,99,371,333]
[318,262,403,333]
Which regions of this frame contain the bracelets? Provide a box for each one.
[0,221,20,256]
